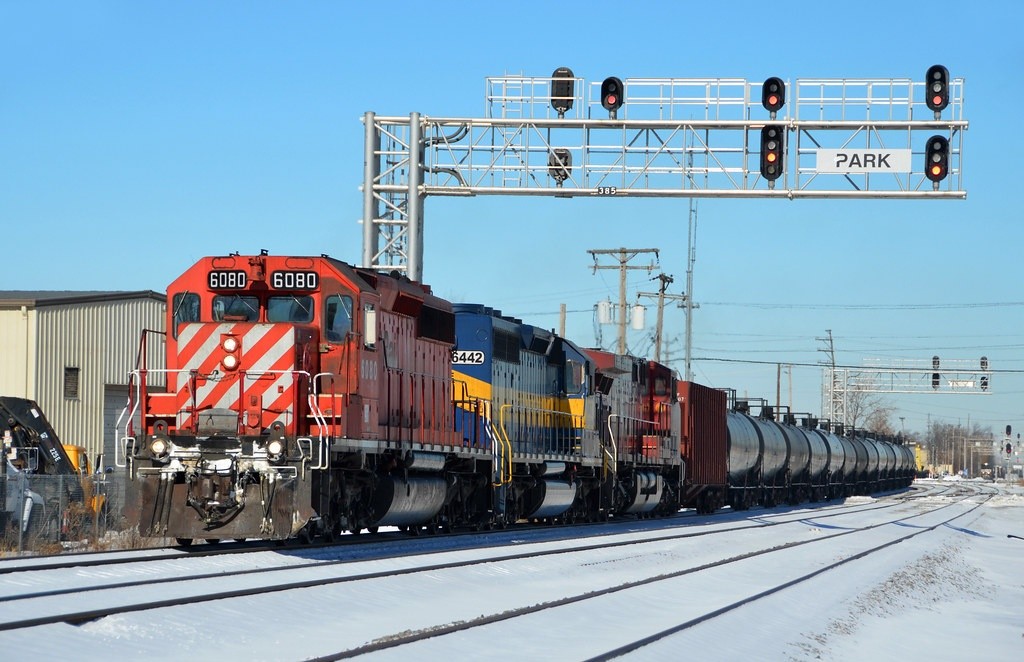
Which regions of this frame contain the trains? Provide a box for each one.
[114,247,920,550]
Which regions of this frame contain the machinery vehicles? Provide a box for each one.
[0,395,114,550]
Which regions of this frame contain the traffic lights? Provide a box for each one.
[759,124,784,181]
[1005,425,1012,436]
[925,64,949,112]
[1006,442,1012,454]
[762,77,786,112]
[925,135,950,182]
[601,76,624,110]
